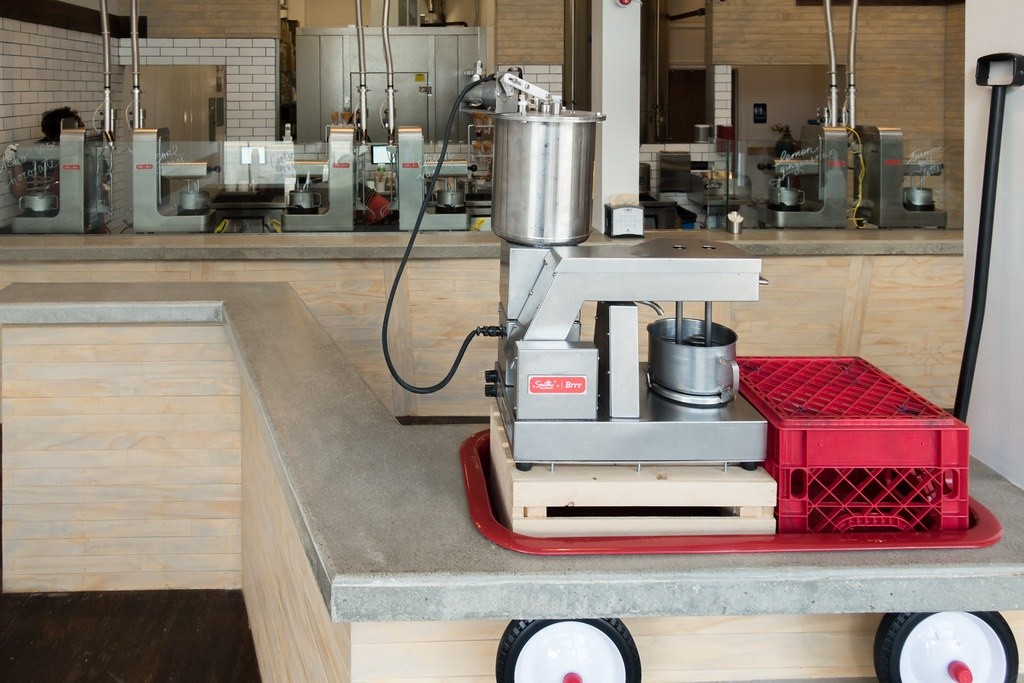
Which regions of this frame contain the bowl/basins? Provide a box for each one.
[904,186,936,208]
[436,190,464,207]
[18,193,59,211]
[645,316,741,396]
[289,190,322,207]
[771,187,806,206]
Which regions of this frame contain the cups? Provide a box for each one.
[424,139,434,162]
[179,193,207,209]
[473,113,483,138]
[448,140,460,160]
[482,141,491,154]
[473,141,480,155]
[727,220,743,234]
[483,116,492,134]
[436,140,447,161]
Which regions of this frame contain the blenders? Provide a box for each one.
[857,126,950,227]
[478,70,768,473]
[11,126,209,234]
[281,124,469,232]
[758,123,850,230]
